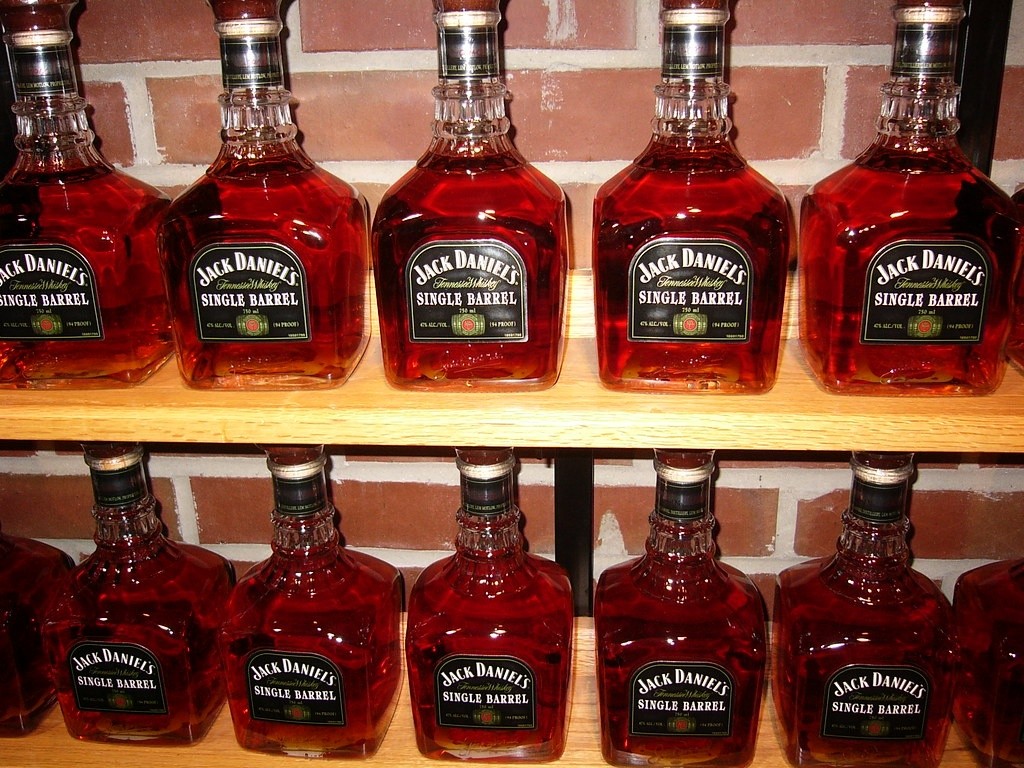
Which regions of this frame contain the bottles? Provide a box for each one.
[799,0,1016,397]
[156,0,368,393]
[405,447,575,764]
[41,444,236,748]
[772,451,952,768]
[951,556,1024,768]
[593,0,788,397]
[593,449,770,768]
[216,444,403,760]
[371,0,570,394]
[0,530,76,738]
[0,0,176,391]
[1009,185,1024,379]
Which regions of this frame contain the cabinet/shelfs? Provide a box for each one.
[0,269,1024,768]
